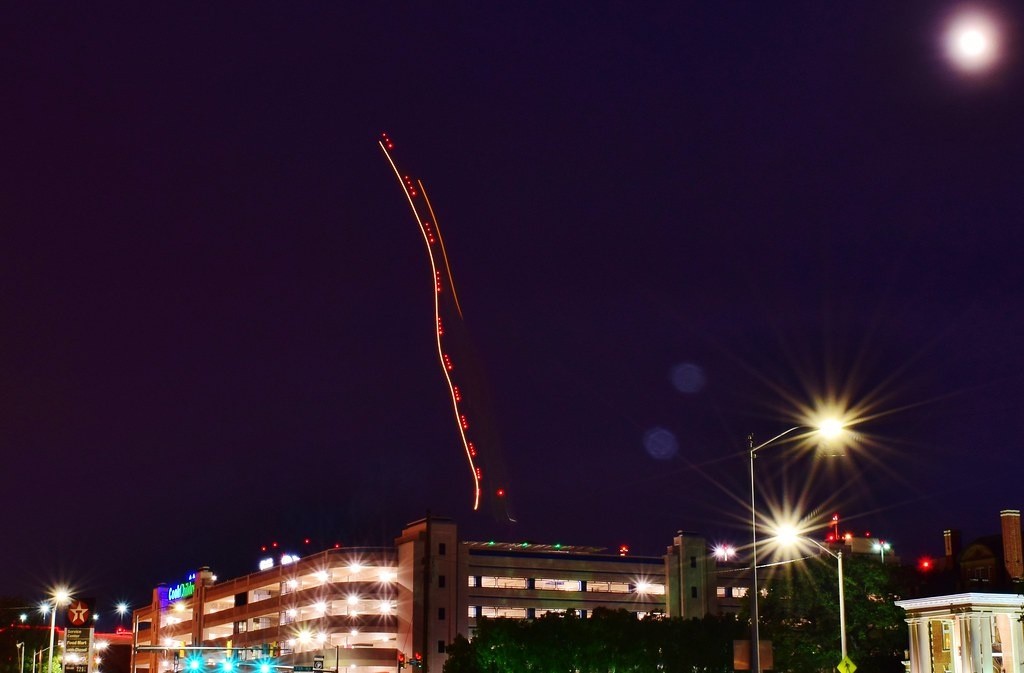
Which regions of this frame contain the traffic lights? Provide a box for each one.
[416,653,423,668]
[260,664,270,673]
[224,662,233,671]
[398,653,405,669]
[190,659,200,668]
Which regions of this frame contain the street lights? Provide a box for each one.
[117,603,127,626]
[33,643,63,673]
[41,604,49,626]
[748,414,845,673]
[301,631,339,673]
[15,641,25,673]
[776,523,848,673]
[47,588,71,673]
